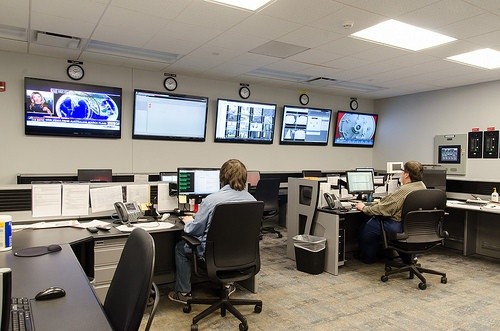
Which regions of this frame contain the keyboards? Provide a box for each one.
[178,216,196,223]
[10,297,36,331]
[352,202,378,207]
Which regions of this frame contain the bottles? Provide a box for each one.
[492,188,498,202]
[0,215,13,251]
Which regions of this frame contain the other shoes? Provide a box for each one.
[353,251,369,263]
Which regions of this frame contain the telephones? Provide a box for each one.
[323,193,343,210]
[114,202,144,224]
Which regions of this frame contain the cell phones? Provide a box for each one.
[87,227,99,232]
[35,287,65,300]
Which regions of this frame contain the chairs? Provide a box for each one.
[102,228,160,331]
[183,201,265,331]
[374,190,447,290]
[251,178,283,241]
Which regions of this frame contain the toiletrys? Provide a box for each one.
[491,187,498,203]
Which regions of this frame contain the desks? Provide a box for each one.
[0,168,500,331]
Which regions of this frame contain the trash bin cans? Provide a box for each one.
[292,234,326,275]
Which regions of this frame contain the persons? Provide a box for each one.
[356,161,428,263]
[28,91,51,114]
[168,159,260,304]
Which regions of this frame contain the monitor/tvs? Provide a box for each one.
[214,98,277,144]
[0,267,12,331]
[160,171,179,185]
[333,111,378,147]
[77,169,112,184]
[438,145,461,164]
[345,171,375,202]
[280,105,332,146]
[23,76,123,138]
[132,90,208,142]
[356,168,375,176]
[177,167,221,204]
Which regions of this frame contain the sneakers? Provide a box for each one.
[225,284,236,297]
[168,291,192,304]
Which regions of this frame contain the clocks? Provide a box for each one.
[163,77,177,91]
[350,100,358,110]
[299,94,309,105]
[239,87,250,99]
[67,64,84,80]
